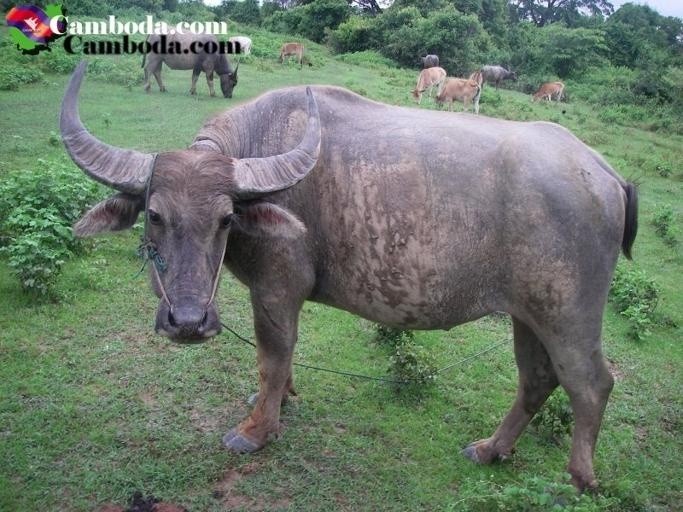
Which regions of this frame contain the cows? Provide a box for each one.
[278,42,304,68]
[410,54,484,115]
[530,81,564,105]
[223,35,253,62]
[58,55,640,498]
[480,65,518,91]
[140,28,240,100]
